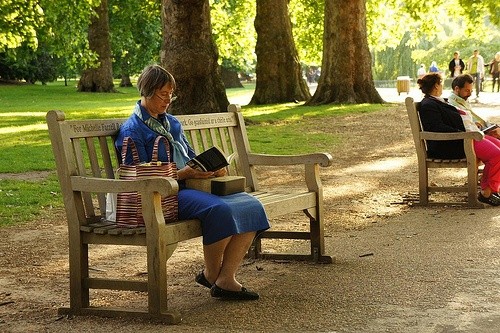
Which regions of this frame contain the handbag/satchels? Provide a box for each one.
[116,136,179,228]
[105,193,117,223]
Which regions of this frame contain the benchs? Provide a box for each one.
[45,102,337,324]
[404,97,500,210]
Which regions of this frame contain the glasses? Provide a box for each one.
[154,91,177,103]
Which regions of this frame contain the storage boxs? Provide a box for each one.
[185,174,247,197]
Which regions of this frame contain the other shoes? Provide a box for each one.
[195,270,213,290]
[477,191,500,206]
[210,283,259,300]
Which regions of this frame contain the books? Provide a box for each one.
[185,145,238,173]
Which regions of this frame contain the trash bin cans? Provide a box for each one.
[396,76,411,97]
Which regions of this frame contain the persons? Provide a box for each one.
[429,61,438,72]
[416,73,500,206]
[489,52,500,92]
[467,50,484,98]
[449,51,465,80]
[417,64,426,79]
[115,65,270,301]
